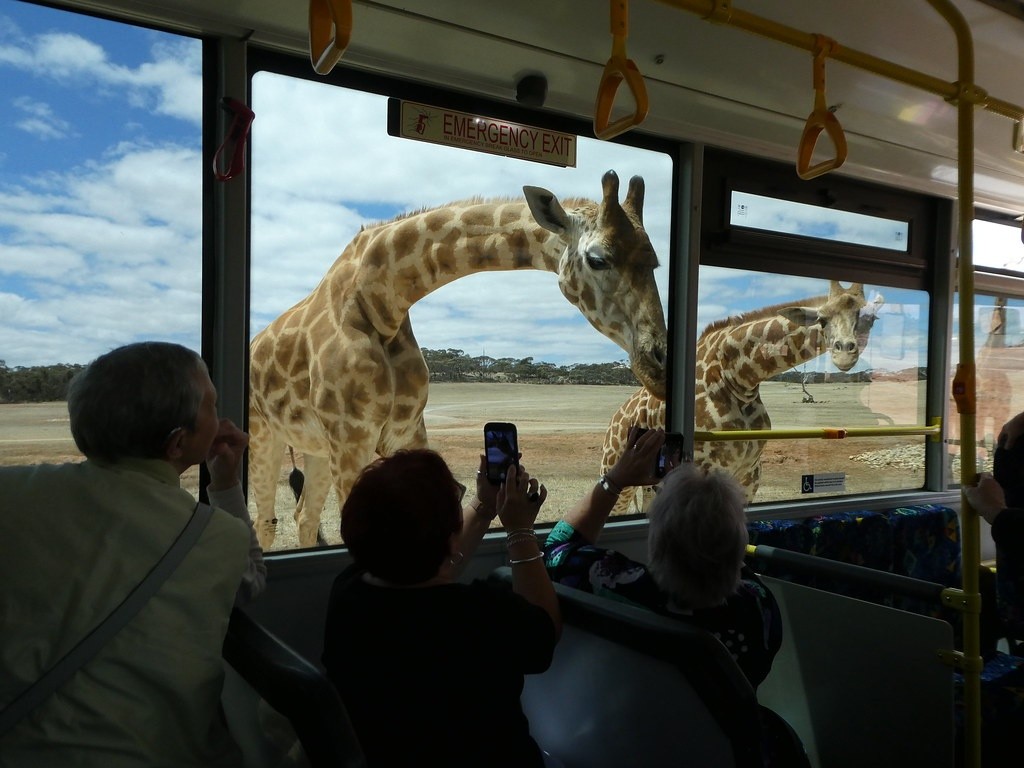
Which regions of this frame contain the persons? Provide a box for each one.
[0,342,270,768]
[298,448,564,768]
[962,471,1023,569]
[543,425,811,768]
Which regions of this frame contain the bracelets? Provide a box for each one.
[599,475,623,496]
[469,497,495,521]
[506,529,545,563]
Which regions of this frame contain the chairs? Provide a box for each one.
[492,566,786,768]
[223,608,369,768]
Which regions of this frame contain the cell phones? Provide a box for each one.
[484,422,518,487]
[655,432,684,480]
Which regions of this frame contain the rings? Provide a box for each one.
[633,444,638,449]
[528,491,539,502]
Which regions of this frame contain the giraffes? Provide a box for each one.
[948,295,1013,487]
[600,281,880,521]
[247,169,667,552]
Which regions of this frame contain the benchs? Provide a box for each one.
[740,503,1024,768]
